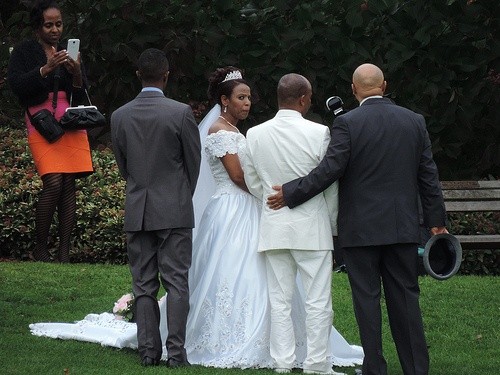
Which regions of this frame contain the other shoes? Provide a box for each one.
[274,368,291,374]
[303,367,348,375]
[167,358,191,368]
[143,357,160,366]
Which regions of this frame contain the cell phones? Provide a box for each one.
[67,38,80,60]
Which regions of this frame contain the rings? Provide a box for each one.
[54,53,57,57]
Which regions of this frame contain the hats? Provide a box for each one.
[422,232,463,280]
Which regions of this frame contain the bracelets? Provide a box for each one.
[40,66,48,78]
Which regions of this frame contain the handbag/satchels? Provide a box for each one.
[32,108,65,143]
[59,86,106,130]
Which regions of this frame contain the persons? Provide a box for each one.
[184,65,271,369]
[111,49,202,368]
[243,73,346,375]
[8,2,94,263]
[267,64,449,375]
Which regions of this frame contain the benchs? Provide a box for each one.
[440,180,500,243]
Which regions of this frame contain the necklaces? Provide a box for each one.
[219,115,241,132]
